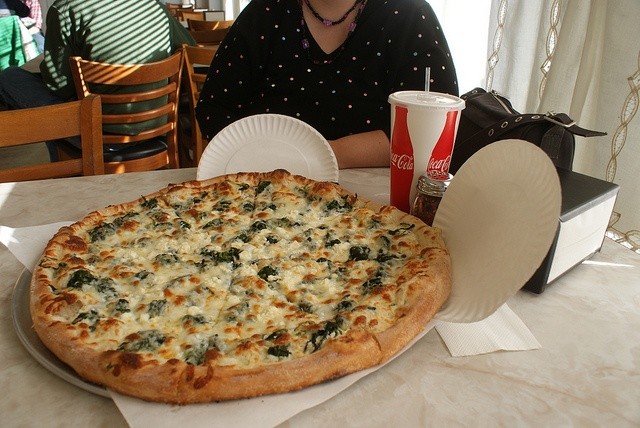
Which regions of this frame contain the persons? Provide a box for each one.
[0,1,196,162]
[12,0,46,54]
[196,0,460,168]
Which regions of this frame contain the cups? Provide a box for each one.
[384,89,468,212]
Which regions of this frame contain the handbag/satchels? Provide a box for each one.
[449,87,607,176]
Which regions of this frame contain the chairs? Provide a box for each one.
[70,44,184,175]
[0,94,104,183]
[166,2,235,47]
[182,41,219,166]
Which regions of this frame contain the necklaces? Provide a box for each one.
[304,0,366,32]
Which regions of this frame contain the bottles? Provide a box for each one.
[409,170,453,224]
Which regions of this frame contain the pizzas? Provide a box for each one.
[29,169,452,403]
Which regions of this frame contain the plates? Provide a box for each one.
[429,138,564,326]
[7,268,112,401]
[194,111,340,187]
